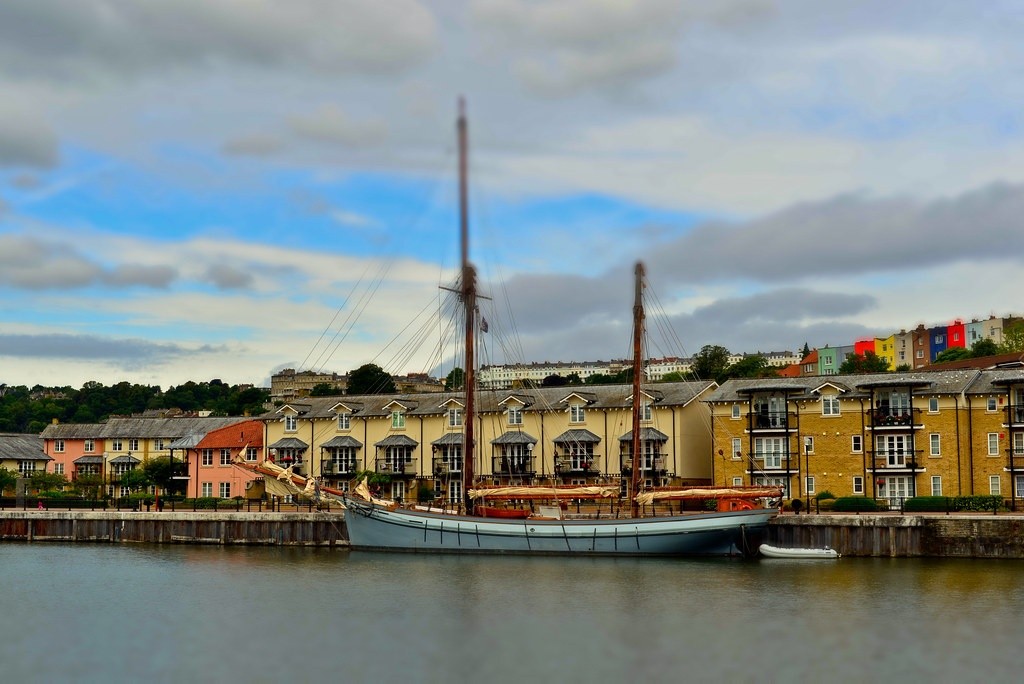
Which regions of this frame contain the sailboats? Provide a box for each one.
[231,95,786,560]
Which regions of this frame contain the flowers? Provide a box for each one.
[877,480,885,485]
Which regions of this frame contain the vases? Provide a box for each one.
[880,484,883,488]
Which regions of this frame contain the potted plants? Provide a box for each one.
[874,406,911,426]
[880,463,885,469]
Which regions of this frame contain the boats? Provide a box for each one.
[759,542,841,559]
